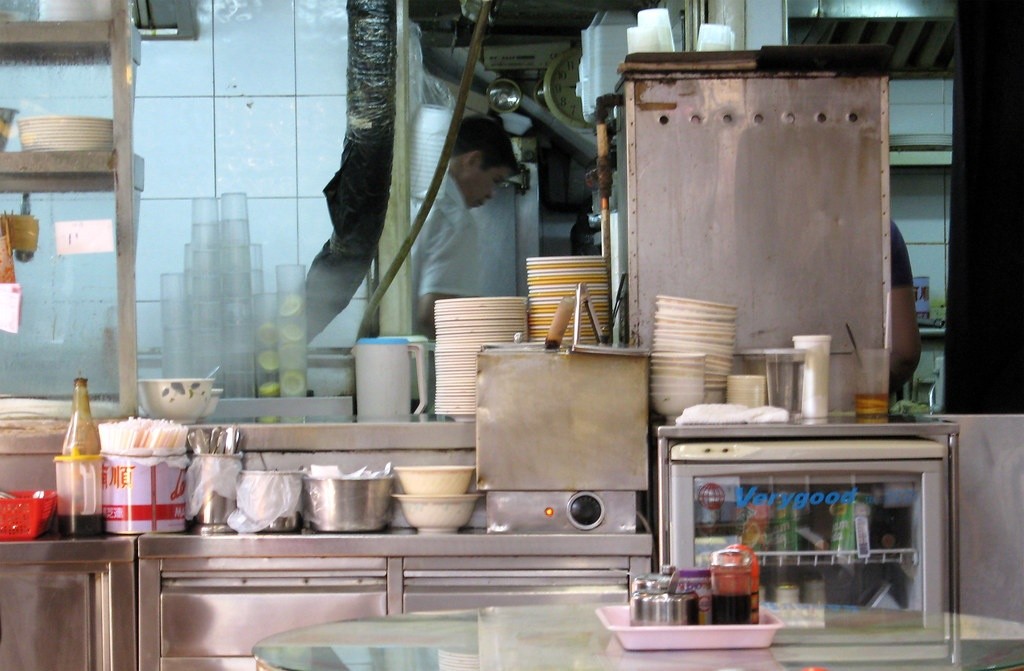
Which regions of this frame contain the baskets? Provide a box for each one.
[0,491,56,541]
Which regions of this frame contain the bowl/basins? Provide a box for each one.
[137,378,214,424]
[410,105,455,202]
[197,389,223,422]
[650,294,738,422]
[243,454,483,532]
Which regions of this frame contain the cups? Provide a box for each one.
[764,335,831,419]
[159,194,308,424]
[627,8,735,55]
[851,348,890,420]
[102,452,241,534]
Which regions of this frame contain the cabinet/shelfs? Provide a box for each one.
[0,0,139,421]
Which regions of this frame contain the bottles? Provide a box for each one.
[53,379,103,537]
[629,543,826,625]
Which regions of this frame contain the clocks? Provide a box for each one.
[543,46,592,130]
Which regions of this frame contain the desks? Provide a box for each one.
[251,600,1024,671]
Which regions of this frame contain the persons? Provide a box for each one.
[372,116,520,339]
[891,217,921,387]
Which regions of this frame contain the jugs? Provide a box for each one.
[350,335,429,417]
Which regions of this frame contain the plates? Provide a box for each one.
[727,374,765,409]
[18,117,113,153]
[434,257,612,421]
[888,134,953,151]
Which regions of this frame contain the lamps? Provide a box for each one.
[485,77,522,113]
